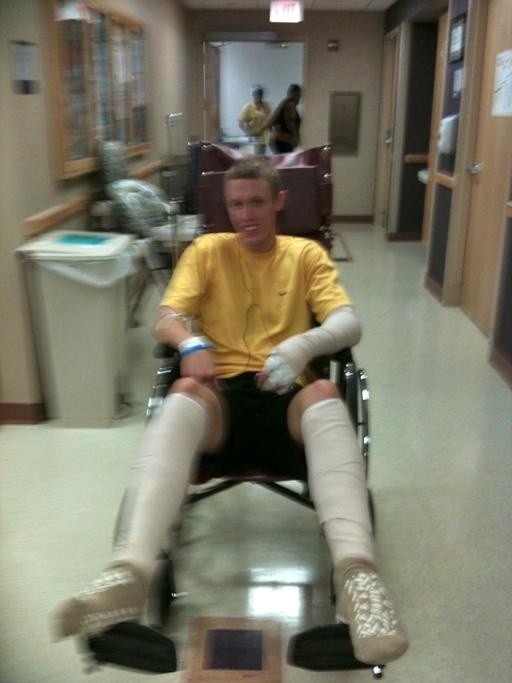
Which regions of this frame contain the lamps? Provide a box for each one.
[267,0,305,24]
[53,0,92,22]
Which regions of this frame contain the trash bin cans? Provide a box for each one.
[13,229,134,426]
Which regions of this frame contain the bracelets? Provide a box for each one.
[176,333,210,355]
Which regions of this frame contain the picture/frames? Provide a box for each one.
[447,10,468,64]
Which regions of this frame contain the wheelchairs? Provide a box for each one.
[82,140,389,683]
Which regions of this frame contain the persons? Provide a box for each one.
[48,154,412,667]
[234,83,273,156]
[248,82,303,154]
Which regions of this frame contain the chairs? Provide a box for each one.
[98,138,206,296]
